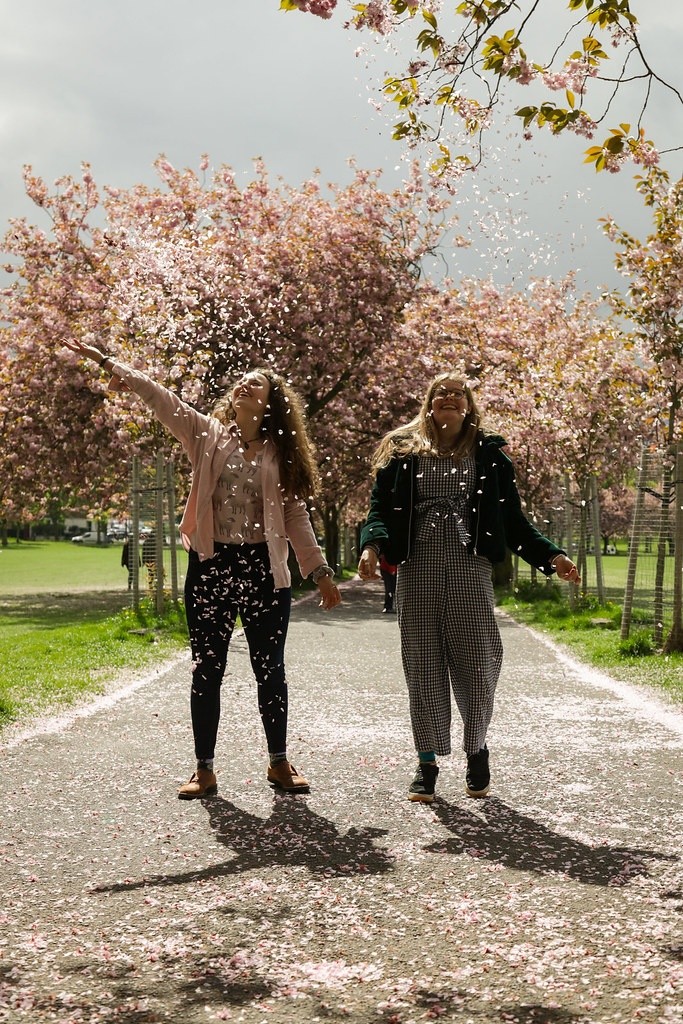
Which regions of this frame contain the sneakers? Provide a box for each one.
[406,763,439,802]
[465,742,490,796]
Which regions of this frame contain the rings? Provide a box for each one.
[332,585,336,589]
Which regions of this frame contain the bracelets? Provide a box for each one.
[312,566,335,584]
[99,358,110,368]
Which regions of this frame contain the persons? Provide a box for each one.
[359,373,583,801]
[59,338,340,803]
[379,552,398,613]
[122,536,142,590]
[142,534,157,591]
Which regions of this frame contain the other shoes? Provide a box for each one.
[179,769,217,798]
[267,760,309,790]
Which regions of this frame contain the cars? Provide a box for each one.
[72,531,105,544]
[105,523,154,543]
